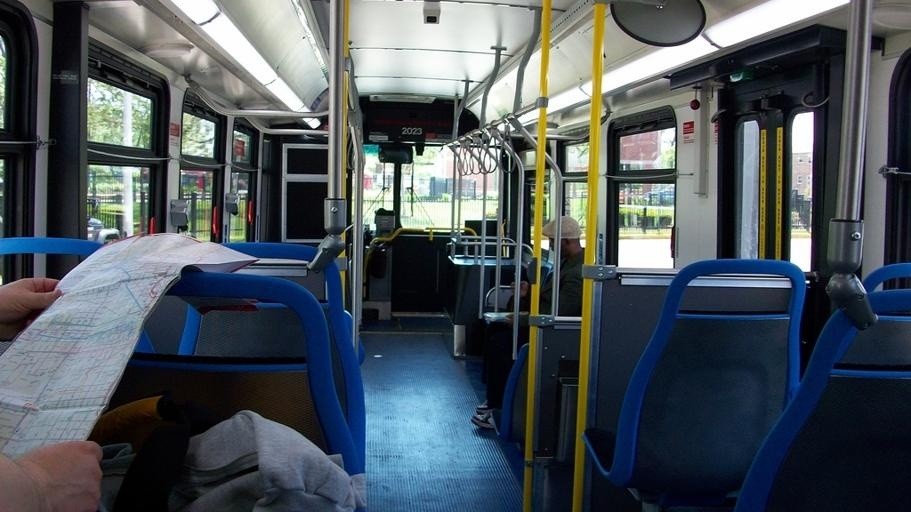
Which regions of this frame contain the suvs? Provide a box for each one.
[0,176,104,249]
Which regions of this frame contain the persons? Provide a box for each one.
[1,278,105,510]
[472,216,586,428]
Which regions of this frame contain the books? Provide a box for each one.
[482,309,531,326]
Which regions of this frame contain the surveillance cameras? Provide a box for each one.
[416,145,424,155]
[423,2,440,24]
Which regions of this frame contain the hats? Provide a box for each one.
[543,216,582,239]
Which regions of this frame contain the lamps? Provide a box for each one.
[610,0,706,47]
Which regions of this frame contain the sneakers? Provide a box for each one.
[471,404,495,428]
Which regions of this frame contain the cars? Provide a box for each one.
[579,183,675,205]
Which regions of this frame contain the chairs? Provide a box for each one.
[0,237,366,477]
[484,258,911,512]
